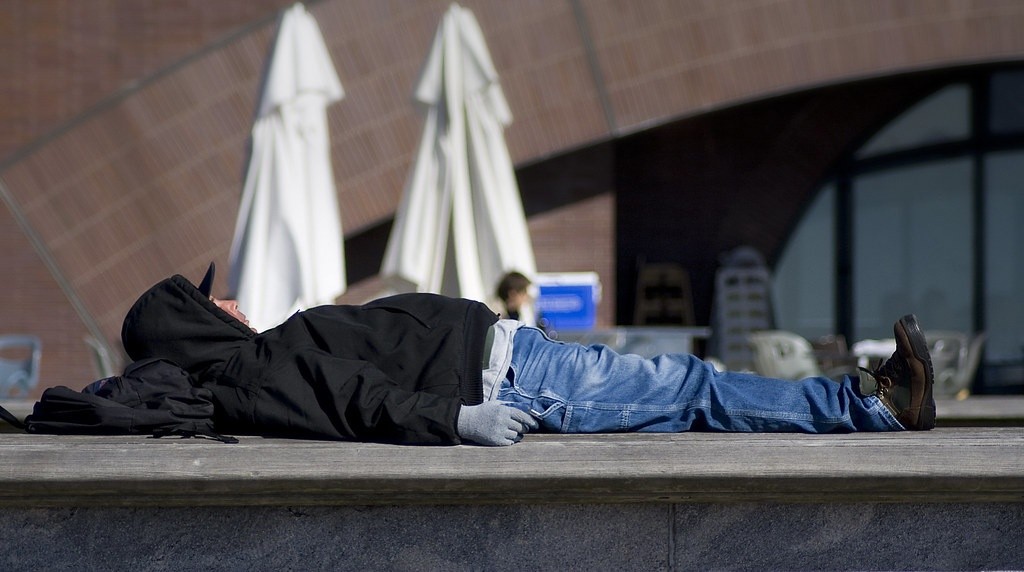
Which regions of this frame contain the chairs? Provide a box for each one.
[1,335,43,398]
[745,324,985,399]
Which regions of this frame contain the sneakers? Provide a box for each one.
[859,314,936,432]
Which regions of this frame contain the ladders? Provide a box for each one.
[716,267,781,372]
[635,267,692,329]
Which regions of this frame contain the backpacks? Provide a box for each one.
[22,358,214,434]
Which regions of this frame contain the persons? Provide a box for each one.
[120,261,937,446]
[486,271,536,328]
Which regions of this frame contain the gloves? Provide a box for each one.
[455,400,539,445]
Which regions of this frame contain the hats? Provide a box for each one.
[197,261,215,297]
